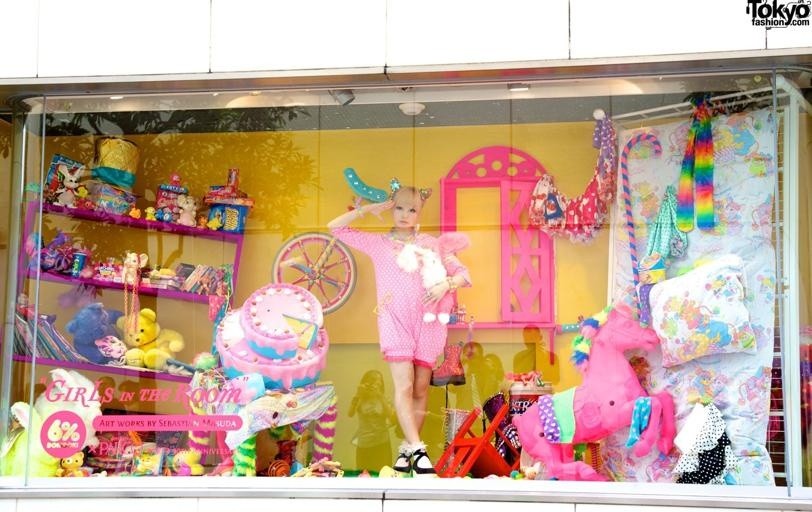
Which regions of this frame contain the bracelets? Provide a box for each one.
[356,204,365,220]
[447,276,455,294]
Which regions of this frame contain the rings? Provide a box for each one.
[427,292,430,297]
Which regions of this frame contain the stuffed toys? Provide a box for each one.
[510,308,678,481]
[17,135,344,479]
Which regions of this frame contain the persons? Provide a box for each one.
[445,324,561,441]
[347,369,405,472]
[326,177,473,476]
